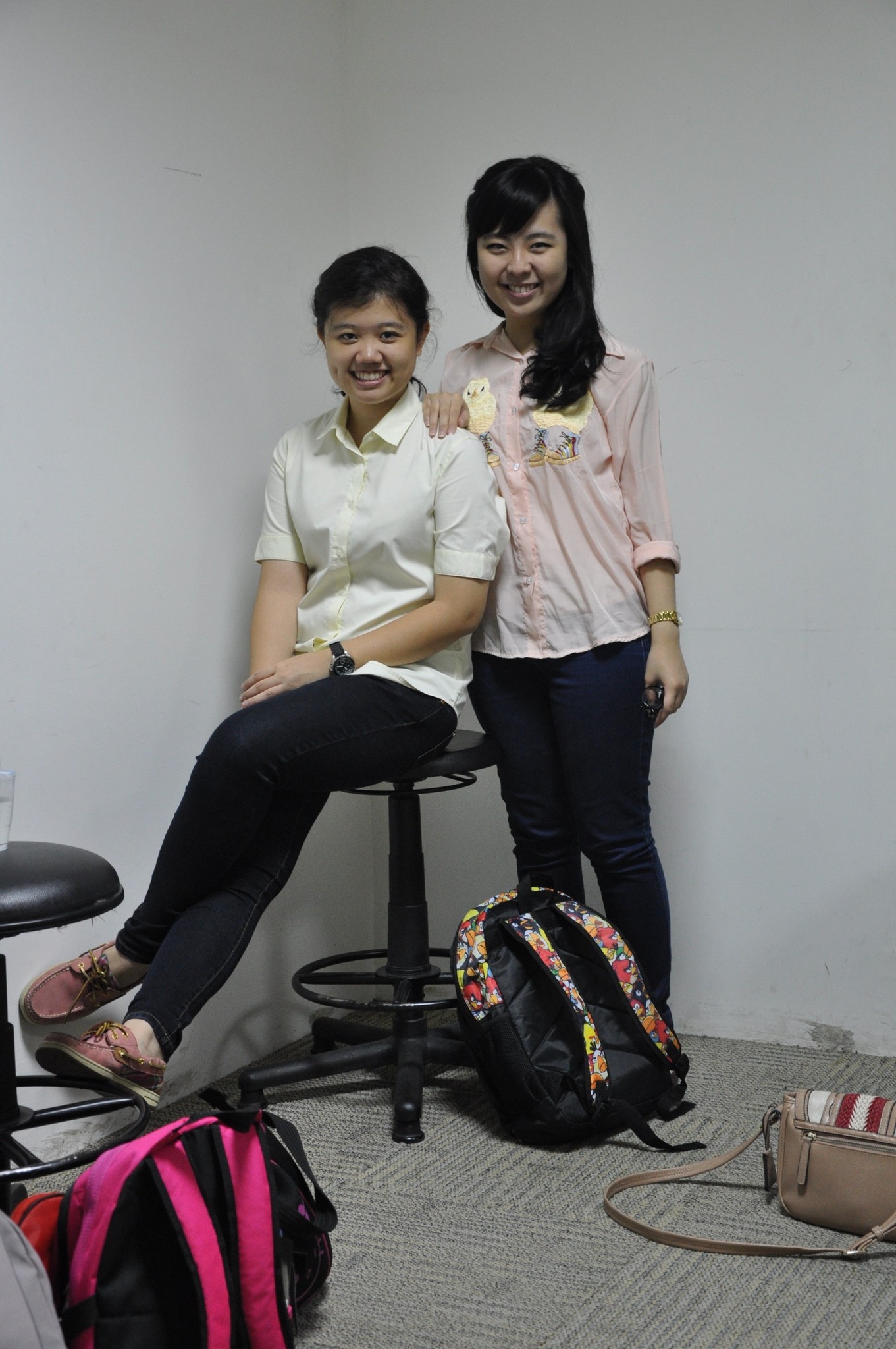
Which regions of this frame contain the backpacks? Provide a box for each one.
[48,1084,340,1349]
[450,872,707,1153]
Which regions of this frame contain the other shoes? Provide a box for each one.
[35,1020,167,1110]
[19,939,147,1025]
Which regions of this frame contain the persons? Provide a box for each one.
[17,242,511,1112]
[421,152,692,1033]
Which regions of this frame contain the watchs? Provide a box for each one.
[329,641,356,677]
[647,610,683,629]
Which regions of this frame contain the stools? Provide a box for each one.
[231,730,492,1144]
[0,840,154,1220]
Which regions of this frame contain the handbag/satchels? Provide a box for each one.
[602,1089,895,1257]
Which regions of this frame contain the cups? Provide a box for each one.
[0,768,16,852]
